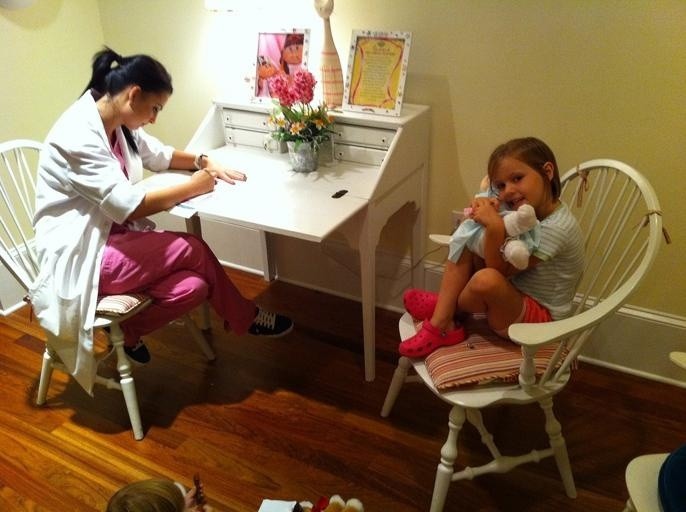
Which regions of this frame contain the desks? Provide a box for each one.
[143,88,432,386]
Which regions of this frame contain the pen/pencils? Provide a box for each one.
[194,472,203,506]
[199,156,203,170]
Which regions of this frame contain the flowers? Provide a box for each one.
[264,70,338,150]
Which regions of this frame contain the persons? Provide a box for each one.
[25,47,295,367]
[105,478,208,511]
[397,137,585,360]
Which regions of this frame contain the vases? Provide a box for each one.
[284,137,323,174]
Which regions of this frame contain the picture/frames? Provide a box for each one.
[341,27,416,118]
[246,23,314,104]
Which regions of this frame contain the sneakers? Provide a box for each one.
[248,305,295,337]
[125,341,151,365]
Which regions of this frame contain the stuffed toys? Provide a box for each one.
[448,173,542,271]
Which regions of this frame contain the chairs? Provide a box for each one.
[1,138,217,443]
[378,154,667,511]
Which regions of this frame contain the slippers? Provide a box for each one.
[403,290,440,320]
[398,318,465,357]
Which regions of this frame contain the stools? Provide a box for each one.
[622,447,685,510]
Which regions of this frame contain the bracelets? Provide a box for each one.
[194,153,208,171]
